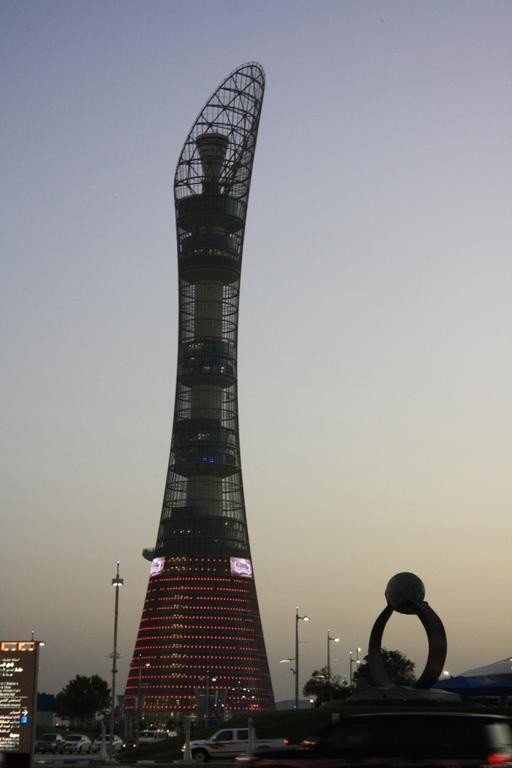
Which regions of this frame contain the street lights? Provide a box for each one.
[231,676,260,717]
[204,669,216,727]
[279,604,310,708]
[316,630,339,685]
[107,561,126,763]
[356,644,362,672]
[346,646,359,685]
[308,697,314,711]
[133,651,151,751]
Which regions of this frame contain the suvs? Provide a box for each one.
[231,708,511,767]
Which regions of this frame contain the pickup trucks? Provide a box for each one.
[178,728,289,761]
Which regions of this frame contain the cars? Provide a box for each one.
[296,734,321,750]
[32,729,177,755]
